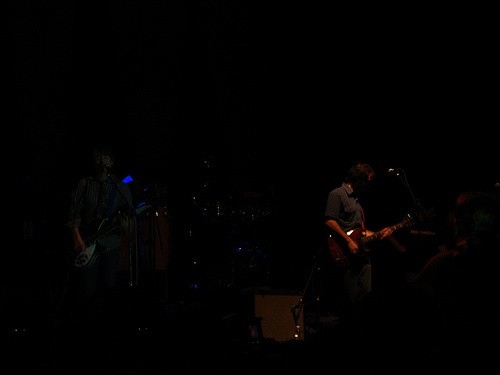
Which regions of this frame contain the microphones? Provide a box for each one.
[105,165,111,177]
[383,167,399,175]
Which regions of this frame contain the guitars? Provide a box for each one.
[329,217,416,261]
[74,201,150,270]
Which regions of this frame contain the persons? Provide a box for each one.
[325,162,391,265]
[65,150,133,295]
